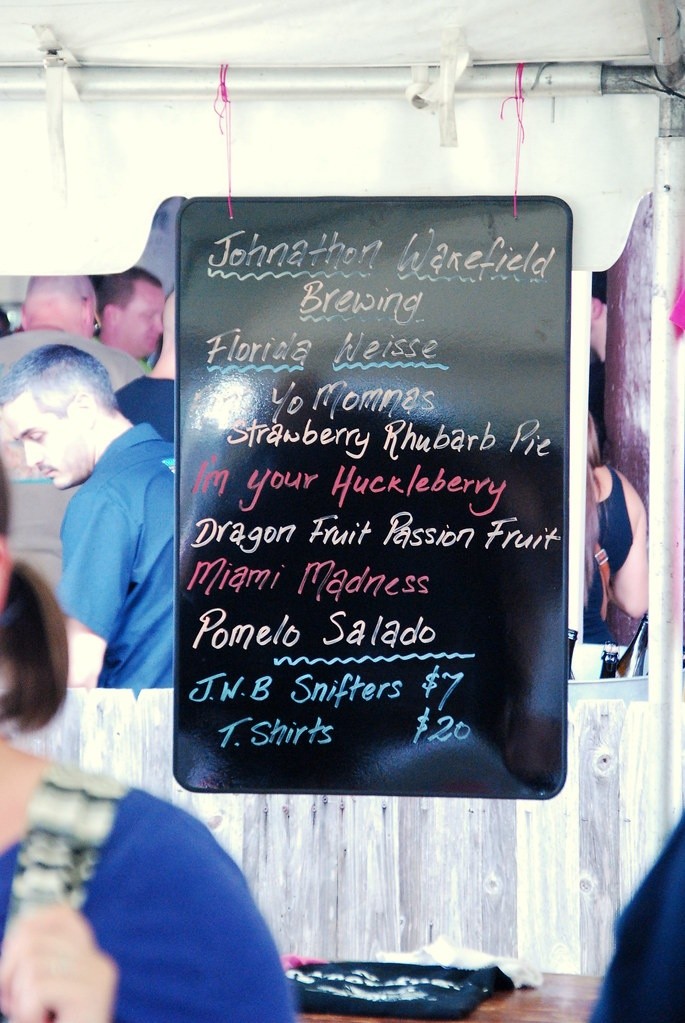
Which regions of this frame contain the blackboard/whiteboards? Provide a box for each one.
[164,195,587,802]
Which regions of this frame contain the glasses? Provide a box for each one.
[92,306,101,334]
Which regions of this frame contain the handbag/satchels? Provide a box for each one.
[285,962,507,1021]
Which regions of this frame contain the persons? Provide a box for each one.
[0,273,148,480]
[110,285,182,443]
[0,345,178,690]
[0,445,301,1023]
[96,266,165,359]
[583,410,649,646]
